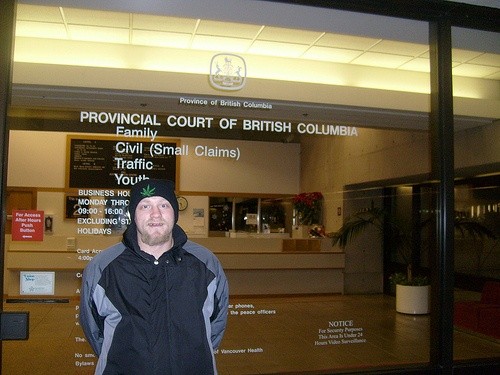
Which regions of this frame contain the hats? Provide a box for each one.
[129,178,179,227]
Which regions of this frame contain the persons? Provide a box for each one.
[80,177,232,375]
